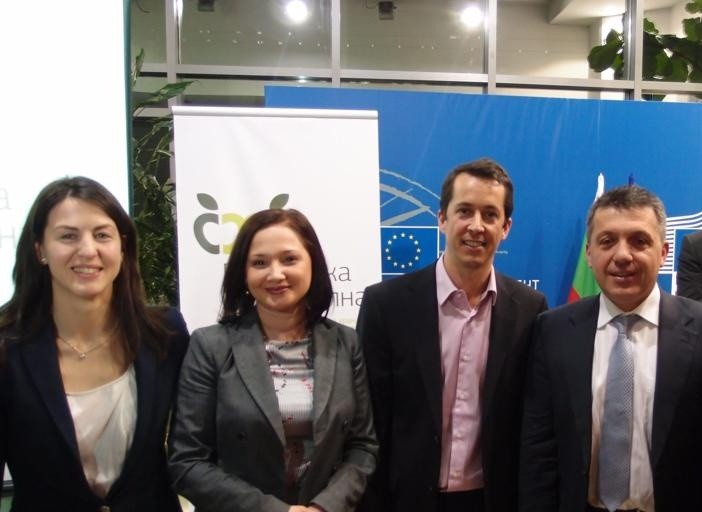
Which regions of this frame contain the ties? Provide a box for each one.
[597,314,643,511]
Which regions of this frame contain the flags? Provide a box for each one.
[567,181,604,305]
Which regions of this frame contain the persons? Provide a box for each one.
[0,173,189,512]
[356,157,550,512]
[163,210,381,512]
[674,229,702,301]
[517,182,702,512]
